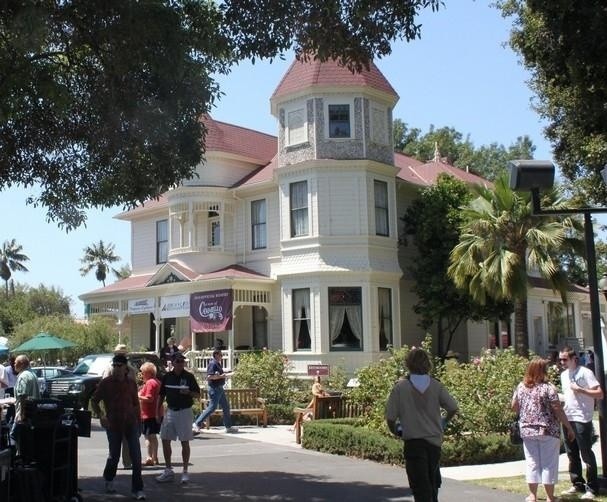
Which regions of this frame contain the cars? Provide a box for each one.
[6,367,68,398]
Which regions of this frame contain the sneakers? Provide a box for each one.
[562,485,586,495]
[225,427,238,433]
[104,479,117,494]
[181,472,191,485]
[131,490,146,501]
[580,491,596,501]
[191,423,200,432]
[155,470,176,483]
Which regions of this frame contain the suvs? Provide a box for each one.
[49,351,167,416]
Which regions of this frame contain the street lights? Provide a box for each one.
[507,158,605,488]
[598,269,607,304]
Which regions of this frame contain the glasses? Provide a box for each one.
[558,358,572,362]
[111,364,125,368]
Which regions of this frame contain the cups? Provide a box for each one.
[4,393,10,399]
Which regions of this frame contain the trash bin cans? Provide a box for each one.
[13,398,78,502]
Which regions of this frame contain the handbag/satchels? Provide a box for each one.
[511,411,523,446]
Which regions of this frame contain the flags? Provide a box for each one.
[188,288,232,333]
[160,293,190,319]
[127,297,155,316]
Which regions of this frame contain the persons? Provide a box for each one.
[0,336,48,459]
[101,343,137,471]
[154,352,200,486]
[555,347,606,502]
[53,359,74,369]
[190,348,238,435]
[384,349,459,501]
[575,349,595,378]
[137,362,162,467]
[511,355,577,501]
[160,337,179,357]
[89,355,148,502]
[213,338,226,350]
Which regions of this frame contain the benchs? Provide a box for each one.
[195,383,272,432]
[289,390,374,448]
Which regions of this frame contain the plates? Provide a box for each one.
[164,385,189,389]
[225,371,234,377]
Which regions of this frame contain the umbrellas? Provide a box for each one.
[9,329,82,380]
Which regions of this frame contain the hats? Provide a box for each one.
[170,353,186,361]
